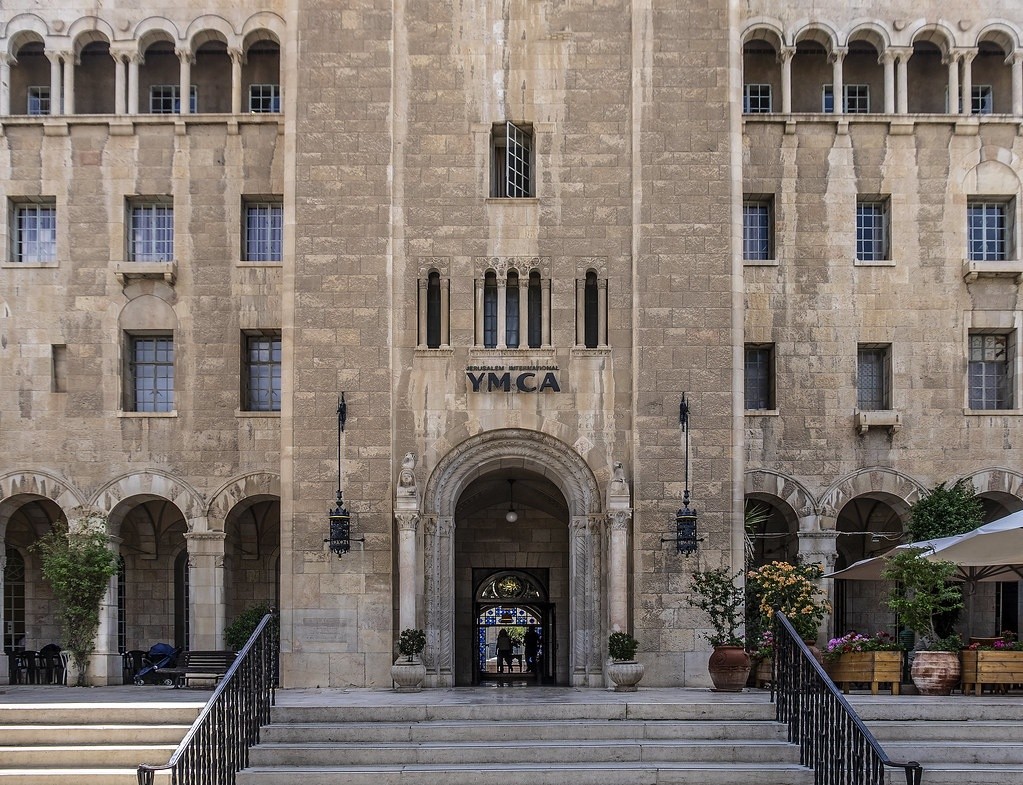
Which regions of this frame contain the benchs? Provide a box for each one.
[155,650,247,691]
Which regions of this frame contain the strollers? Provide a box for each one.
[132,641,182,686]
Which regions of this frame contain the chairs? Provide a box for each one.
[6,644,151,685]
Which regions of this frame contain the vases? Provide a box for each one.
[786,640,823,693]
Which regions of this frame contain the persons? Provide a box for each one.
[522,624,540,672]
[397,469,418,497]
[495,628,514,673]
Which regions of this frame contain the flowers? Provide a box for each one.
[969,630,1023,651]
[749,630,774,661]
[820,631,906,664]
[747,560,834,641]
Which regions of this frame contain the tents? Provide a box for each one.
[816,527,1023,652]
[904,507,1023,572]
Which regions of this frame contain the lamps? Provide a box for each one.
[506,479,518,522]
[390,628,427,694]
[322,392,365,560]
[661,392,705,559]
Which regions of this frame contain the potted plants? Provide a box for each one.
[684,565,752,694]
[877,542,967,696]
[607,631,645,692]
